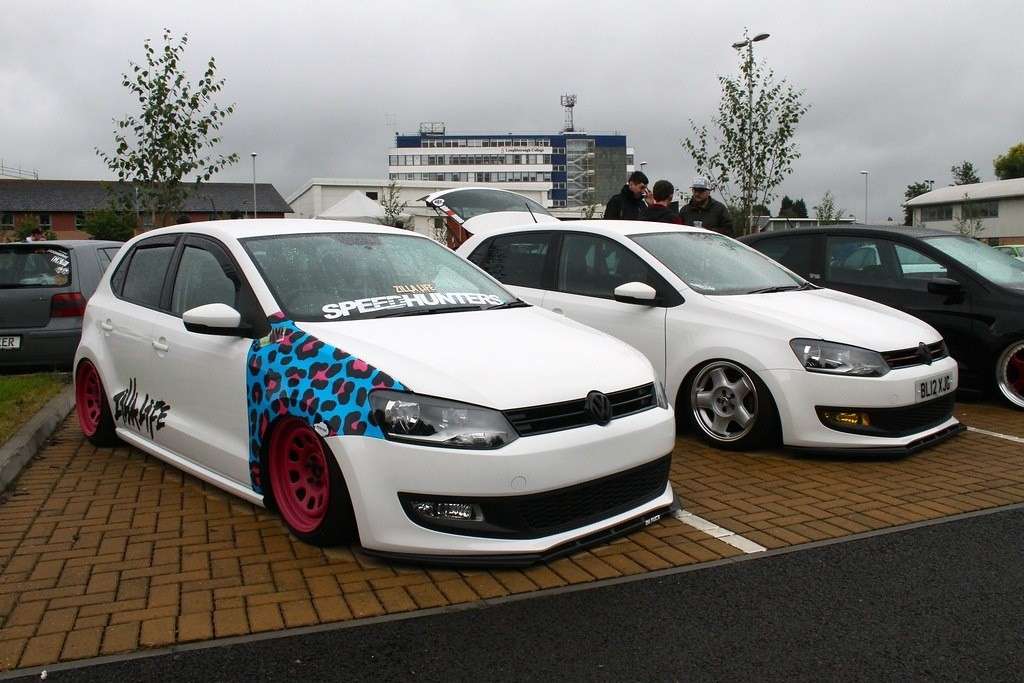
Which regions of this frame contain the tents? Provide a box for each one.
[311,189,415,231]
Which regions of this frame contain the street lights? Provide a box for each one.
[732,33,773,233]
[860,170,868,224]
[640,162,647,171]
[250,152,258,219]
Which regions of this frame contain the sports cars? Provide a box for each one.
[419,188,970,460]
[74,216,678,569]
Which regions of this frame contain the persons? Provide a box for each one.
[603,170,654,271]
[18,227,43,279]
[395,220,404,228]
[633,180,684,225]
[680,176,735,236]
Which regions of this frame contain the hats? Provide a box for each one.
[690,177,712,190]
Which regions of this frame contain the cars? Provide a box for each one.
[734,223,1024,412]
[991,243,1024,268]
[0,240,132,375]
[830,241,949,275]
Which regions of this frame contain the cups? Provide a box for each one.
[694,221,703,228]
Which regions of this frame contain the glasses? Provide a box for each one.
[691,188,708,193]
